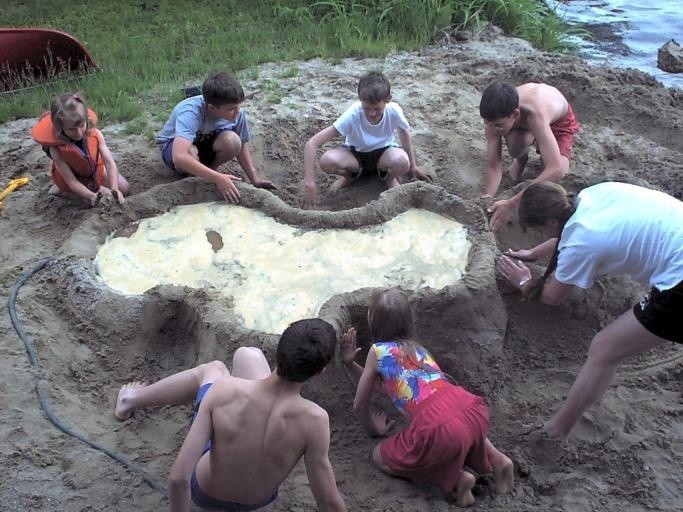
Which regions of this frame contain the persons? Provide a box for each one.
[340,289,516,507]
[112,317,348,511]
[296,71,436,210]
[155,71,280,205]
[495,180,683,446]
[30,90,131,210]
[471,82,581,235]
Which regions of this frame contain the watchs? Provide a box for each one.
[518,278,530,290]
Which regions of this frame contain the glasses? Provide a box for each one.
[483,119,512,132]
